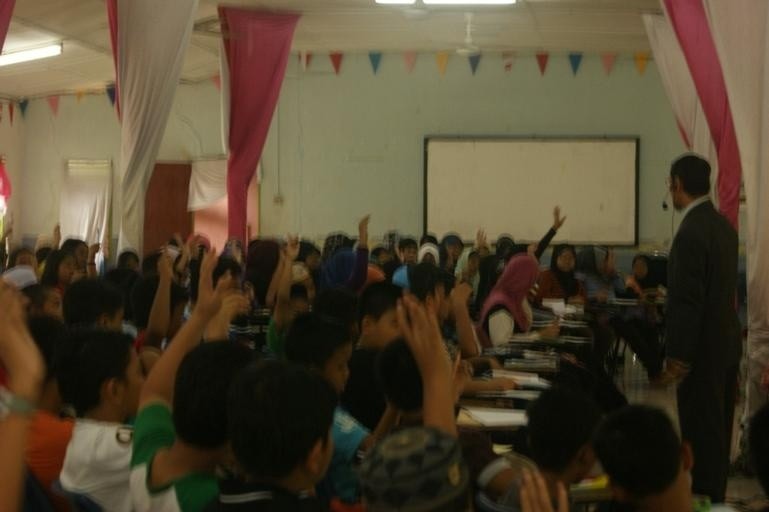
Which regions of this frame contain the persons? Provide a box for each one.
[656,150,745,506]
[1,203,695,512]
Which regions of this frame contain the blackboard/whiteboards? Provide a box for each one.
[423,135,640,247]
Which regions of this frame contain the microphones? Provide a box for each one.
[662,187,674,211]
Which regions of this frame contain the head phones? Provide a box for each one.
[670,162,709,192]
